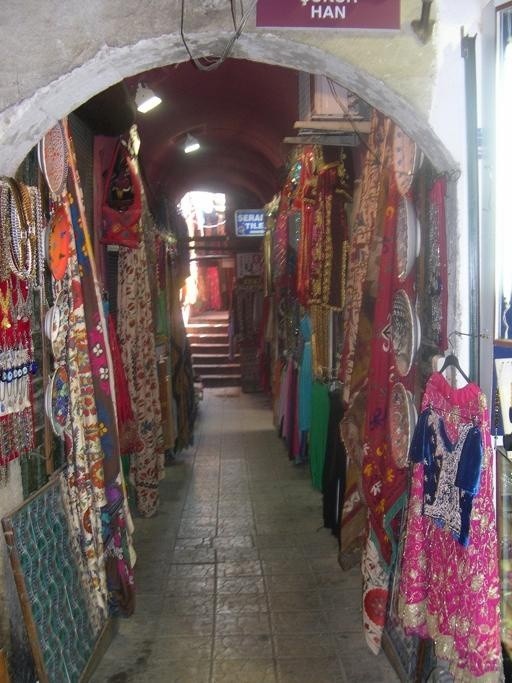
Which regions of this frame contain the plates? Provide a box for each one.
[391,289,422,376]
[37,121,69,195]
[387,381,418,468]
[392,125,424,196]
[44,366,69,435]
[42,205,70,281]
[395,196,422,281]
[43,289,71,361]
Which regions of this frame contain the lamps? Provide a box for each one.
[134,76,161,114]
[185,132,199,153]
